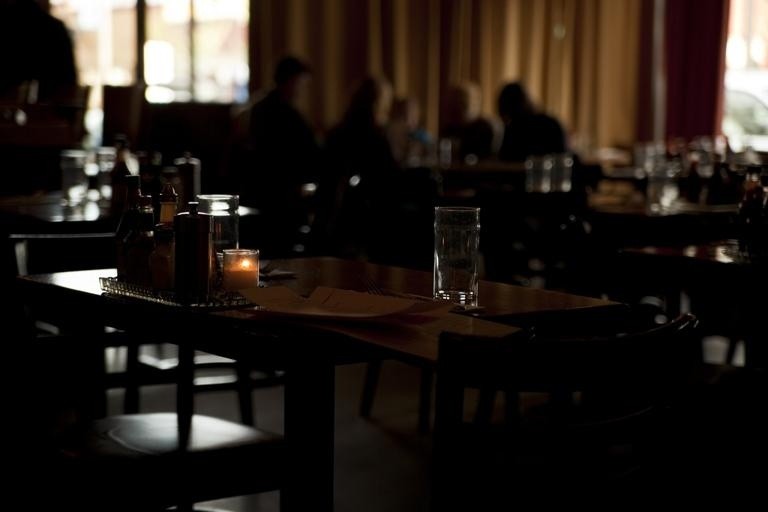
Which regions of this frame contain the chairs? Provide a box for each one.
[0,184,768,512]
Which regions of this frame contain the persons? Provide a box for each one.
[0,1,78,198]
[238,56,567,213]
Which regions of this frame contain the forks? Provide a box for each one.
[364,278,385,296]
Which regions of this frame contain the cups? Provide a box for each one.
[434,206,480,306]
[196,195,239,267]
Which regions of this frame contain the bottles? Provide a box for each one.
[118,157,201,292]
